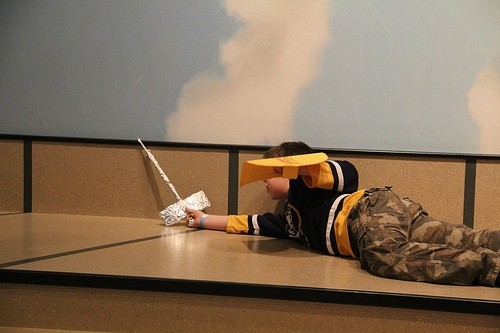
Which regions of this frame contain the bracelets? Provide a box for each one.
[199,212,208,229]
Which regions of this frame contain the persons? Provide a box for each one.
[185,141,500,288]
